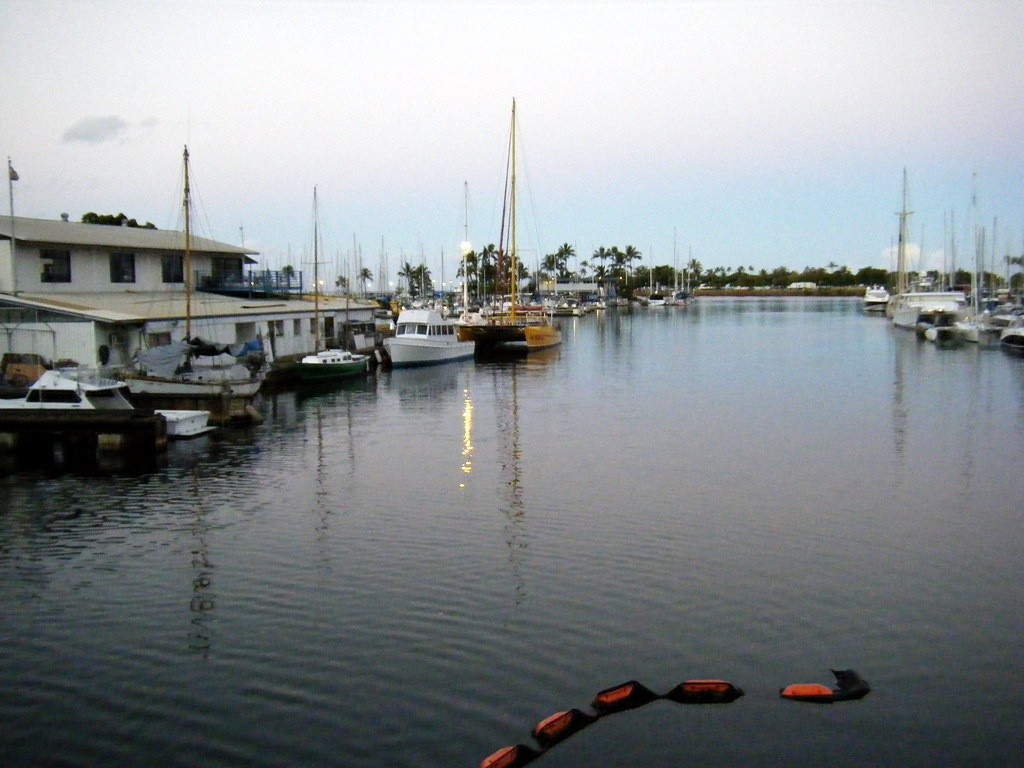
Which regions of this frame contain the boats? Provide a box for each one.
[0,361,218,437]
[864,284,889,304]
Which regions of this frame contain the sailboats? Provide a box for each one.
[289,185,373,380]
[380,91,692,369]
[123,144,267,399]
[884,164,1024,352]
